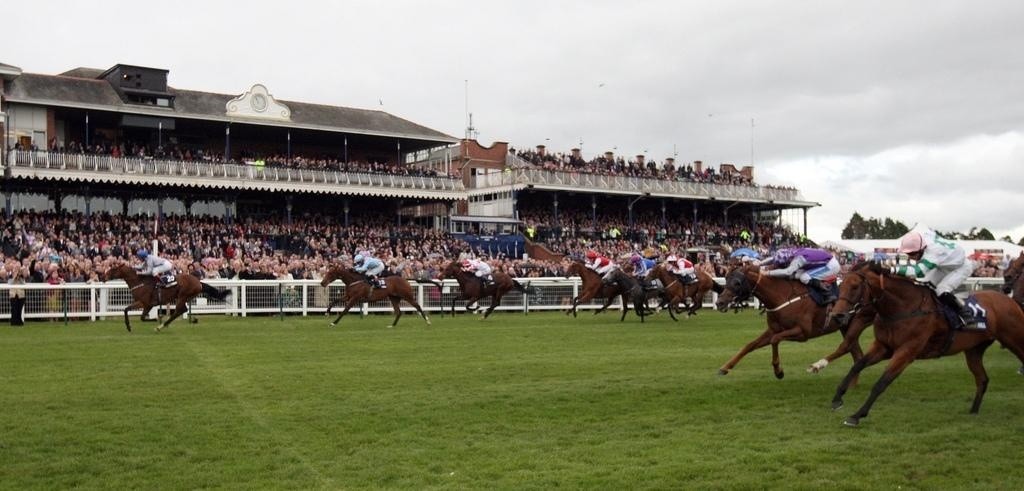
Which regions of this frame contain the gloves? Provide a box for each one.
[759,270,768,277]
[870,263,890,276]
[752,260,761,267]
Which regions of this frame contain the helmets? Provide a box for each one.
[353,255,363,267]
[136,250,147,258]
[631,256,641,263]
[462,260,470,266]
[773,248,793,265]
[742,256,749,262]
[587,252,595,259]
[666,255,677,263]
[900,232,926,254]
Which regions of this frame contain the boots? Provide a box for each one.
[938,293,976,330]
[480,274,488,289]
[154,275,165,289]
[807,279,838,304]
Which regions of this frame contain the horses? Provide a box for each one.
[438,261,536,317]
[318,260,433,329]
[827,259,1023,428]
[560,260,726,322]
[714,262,868,389]
[101,262,232,333]
[999,249,1023,375]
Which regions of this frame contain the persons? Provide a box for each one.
[2,208,1000,328]
[511,148,795,192]
[13,134,437,178]
